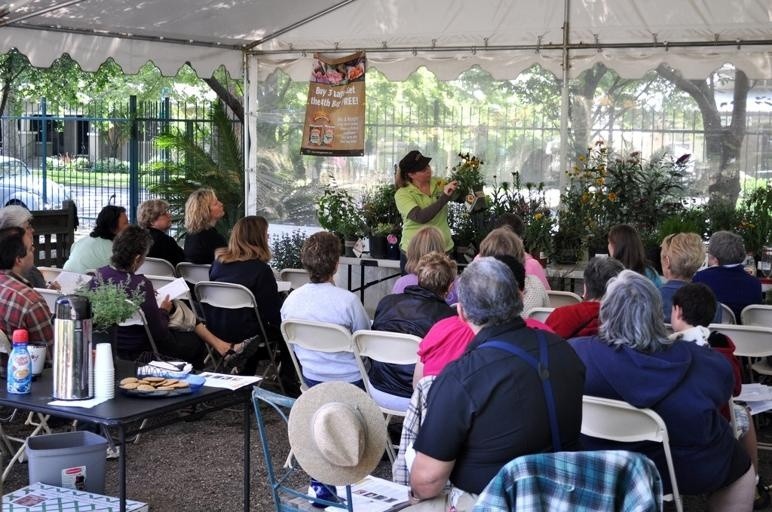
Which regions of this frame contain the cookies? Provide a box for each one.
[120,377,191,397]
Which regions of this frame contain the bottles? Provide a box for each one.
[6,329,34,393]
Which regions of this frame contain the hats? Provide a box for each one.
[710,229,747,261]
[288,379,388,487]
[399,150,432,175]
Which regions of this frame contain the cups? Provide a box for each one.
[95,343,115,400]
[308,478,337,508]
[26,343,48,376]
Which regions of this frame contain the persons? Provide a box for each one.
[394,151,459,274]
[0,189,282,377]
[279,213,763,512]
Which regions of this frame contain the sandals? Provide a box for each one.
[222,334,262,371]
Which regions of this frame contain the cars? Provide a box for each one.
[1,149,78,229]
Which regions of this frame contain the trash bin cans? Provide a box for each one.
[25,431,109,494]
